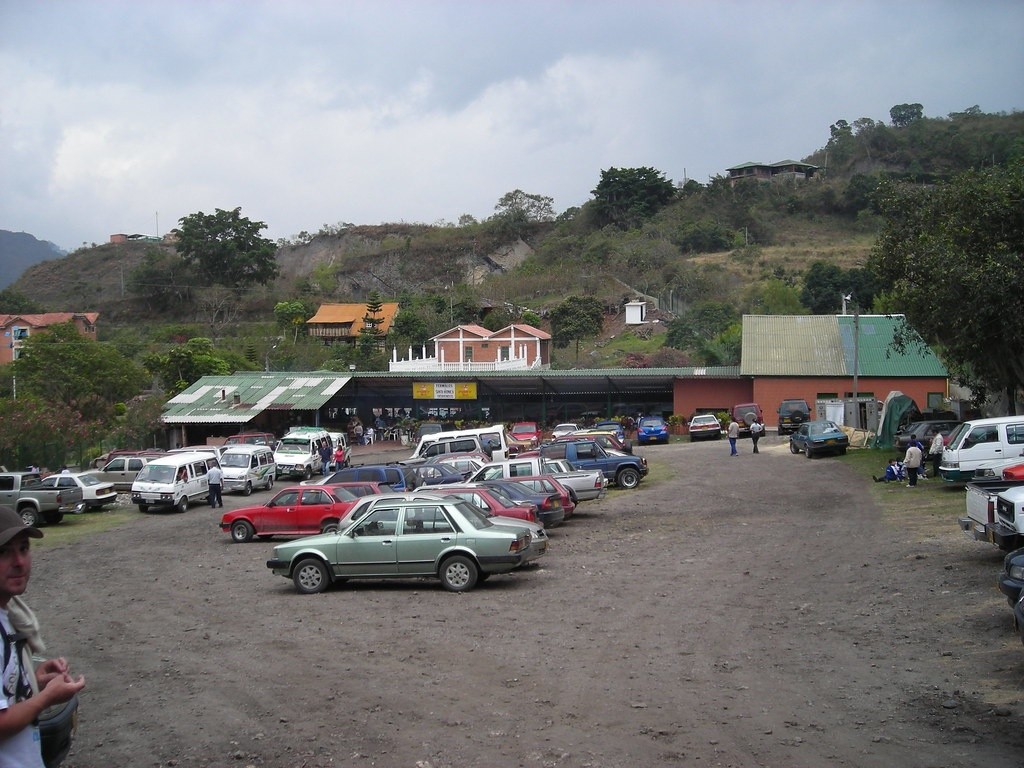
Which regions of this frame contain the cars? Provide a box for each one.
[551,423,581,442]
[511,421,544,448]
[85,456,159,493]
[893,419,965,462]
[687,414,722,442]
[218,484,359,544]
[957,456,1024,645]
[39,469,118,515]
[88,433,286,469]
[788,419,850,459]
[267,498,533,595]
[632,416,670,445]
[314,424,649,535]
[594,420,626,442]
[335,491,549,567]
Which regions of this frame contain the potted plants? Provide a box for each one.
[396,416,411,446]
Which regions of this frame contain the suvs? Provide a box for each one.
[729,403,766,437]
[776,398,812,436]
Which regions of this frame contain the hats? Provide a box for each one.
[0,506,43,548]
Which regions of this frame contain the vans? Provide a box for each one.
[218,445,276,496]
[939,415,1024,483]
[131,451,224,514]
[273,427,334,481]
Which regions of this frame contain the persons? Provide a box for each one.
[318,441,333,476]
[178,469,187,480]
[0,507,85,768]
[929,427,944,477]
[872,434,924,484]
[348,415,385,446]
[207,462,224,508]
[332,445,344,471]
[903,441,921,488]
[62,466,69,473]
[726,417,739,456]
[750,419,763,453]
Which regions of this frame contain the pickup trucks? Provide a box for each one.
[328,431,352,470]
[0,471,83,528]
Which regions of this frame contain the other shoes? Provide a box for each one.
[906,485,914,488]
[873,476,878,482]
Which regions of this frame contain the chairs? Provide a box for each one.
[406,518,417,534]
[363,428,383,445]
[388,429,398,442]
